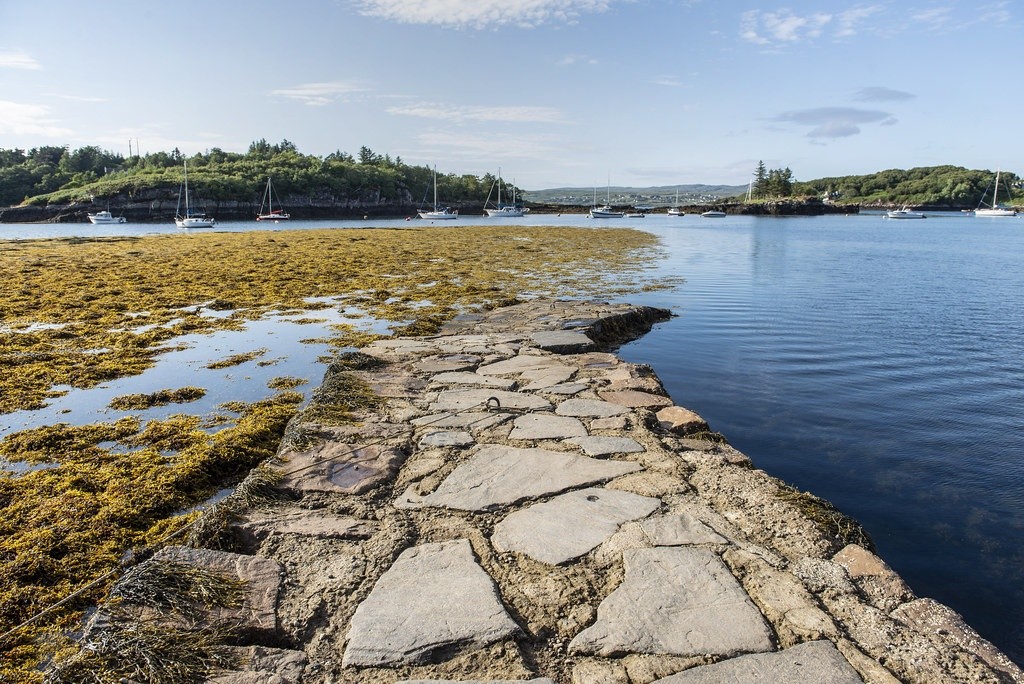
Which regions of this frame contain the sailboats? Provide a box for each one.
[973,168,1021,216]
[483,167,530,217]
[254,176,290,221]
[590,170,625,218]
[173,161,215,228]
[668,189,685,215]
[417,164,458,219]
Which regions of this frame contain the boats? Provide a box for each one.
[701,210,726,217]
[87,200,128,224]
[887,201,923,218]
[627,213,645,218]
